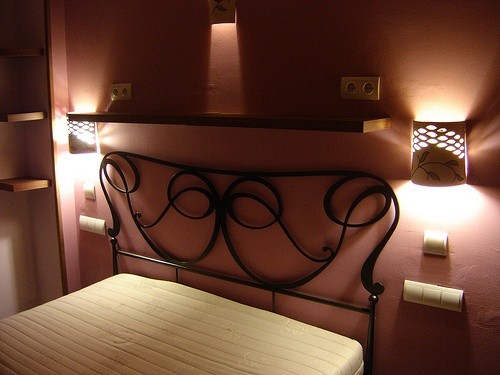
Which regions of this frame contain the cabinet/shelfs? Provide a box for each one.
[0,47,52,192]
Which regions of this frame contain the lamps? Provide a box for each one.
[208,0,237,24]
[67,112,97,156]
[409,119,470,187]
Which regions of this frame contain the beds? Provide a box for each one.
[0,274,371,375]
